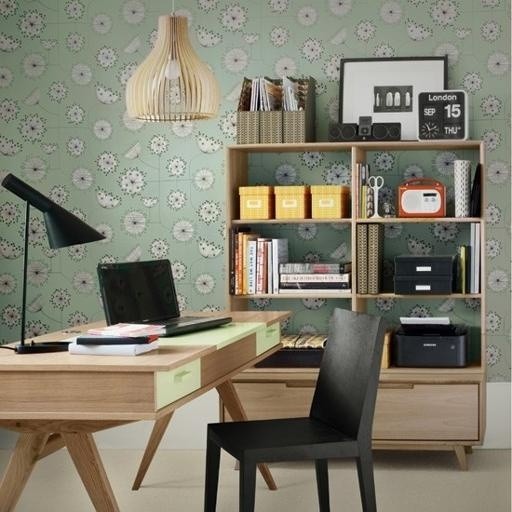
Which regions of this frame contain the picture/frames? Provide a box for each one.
[338,54,451,142]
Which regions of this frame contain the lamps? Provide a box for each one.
[124,0,222,123]
[0,171,108,355]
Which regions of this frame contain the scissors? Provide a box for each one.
[367,176,384,218]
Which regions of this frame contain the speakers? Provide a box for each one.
[328,122,401,142]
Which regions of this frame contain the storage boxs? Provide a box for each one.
[392,253,457,296]
[238,184,350,221]
[394,329,471,369]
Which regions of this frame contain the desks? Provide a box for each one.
[1,309,293,512]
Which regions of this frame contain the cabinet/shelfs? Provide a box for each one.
[222,136,487,470]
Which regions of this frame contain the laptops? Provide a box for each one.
[97,259,232,337]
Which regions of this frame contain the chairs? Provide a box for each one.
[201,305,389,512]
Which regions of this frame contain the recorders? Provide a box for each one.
[398,177,447,217]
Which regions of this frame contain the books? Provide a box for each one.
[356,162,371,218]
[459,222,480,294]
[68,323,166,357]
[230,227,352,296]
[355,222,368,295]
[367,223,385,294]
